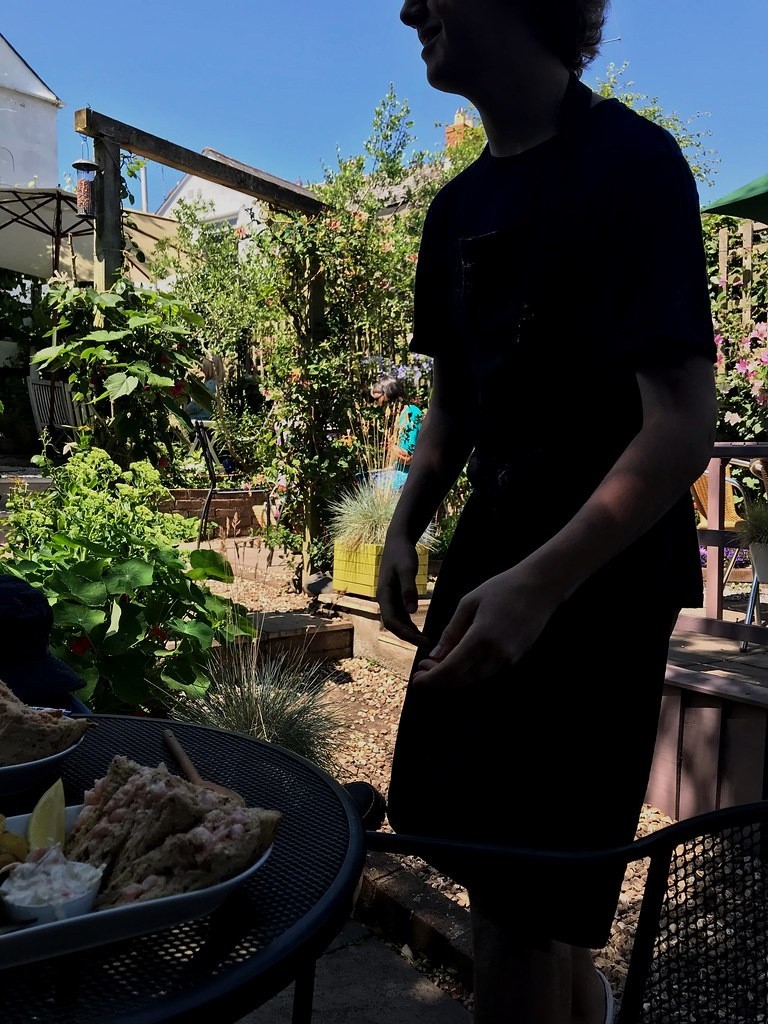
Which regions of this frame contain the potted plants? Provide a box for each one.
[322,478,442,602]
[726,496,768,583]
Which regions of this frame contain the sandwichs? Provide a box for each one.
[63,753,279,909]
[0,679,97,768]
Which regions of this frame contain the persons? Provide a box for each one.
[371,377,424,474]
[375,1,719,1024]
[185,354,226,455]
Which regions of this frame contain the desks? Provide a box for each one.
[0,713,367,1024]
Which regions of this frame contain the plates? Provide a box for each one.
[0,715,85,773]
[0,805,273,944]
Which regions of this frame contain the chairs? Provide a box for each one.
[290,801,768,1024]
[26,376,76,440]
[194,420,270,550]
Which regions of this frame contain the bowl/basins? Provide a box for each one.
[0,862,102,926]
